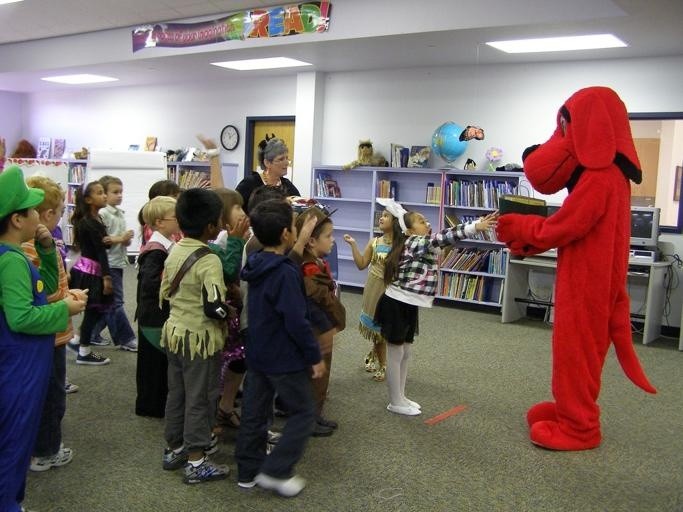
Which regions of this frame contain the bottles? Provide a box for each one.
[426,183,434,204]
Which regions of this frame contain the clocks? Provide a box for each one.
[221,125,240,151]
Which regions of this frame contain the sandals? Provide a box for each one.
[364,353,376,373]
[374,364,386,382]
[215,407,241,429]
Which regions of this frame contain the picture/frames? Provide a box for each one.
[673,166,683,201]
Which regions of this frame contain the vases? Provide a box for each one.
[488,162,496,172]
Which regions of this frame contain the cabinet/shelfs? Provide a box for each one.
[438,172,533,323]
[63,160,86,246]
[166,163,238,191]
[312,166,444,298]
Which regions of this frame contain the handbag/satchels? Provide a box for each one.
[499,194,548,217]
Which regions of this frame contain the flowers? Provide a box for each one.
[486,145,502,162]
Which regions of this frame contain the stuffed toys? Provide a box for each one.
[497,85,657,451]
[342,140,388,171]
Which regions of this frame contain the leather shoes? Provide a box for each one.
[310,421,333,437]
[318,416,337,428]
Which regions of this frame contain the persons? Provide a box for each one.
[373,206,499,414]
[131,135,345,496]
[343,208,397,381]
[1,166,138,511]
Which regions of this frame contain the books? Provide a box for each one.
[391,144,433,168]
[379,181,398,200]
[427,187,442,205]
[36,137,211,245]
[440,180,517,304]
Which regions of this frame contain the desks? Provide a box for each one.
[501,249,672,345]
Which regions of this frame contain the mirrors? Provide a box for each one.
[629,112,683,234]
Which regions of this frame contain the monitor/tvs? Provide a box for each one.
[628,205,661,249]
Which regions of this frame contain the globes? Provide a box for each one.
[432,121,468,170]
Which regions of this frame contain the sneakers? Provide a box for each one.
[238,481,256,488]
[255,473,306,497]
[163,444,187,470]
[119,340,138,352]
[403,397,421,409]
[76,351,111,365]
[205,434,217,455]
[29,448,73,471]
[272,402,287,418]
[386,402,421,415]
[183,452,230,484]
[65,379,78,394]
[90,335,111,346]
[66,341,79,354]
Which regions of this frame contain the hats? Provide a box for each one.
[295,206,338,238]
[0,165,45,218]
